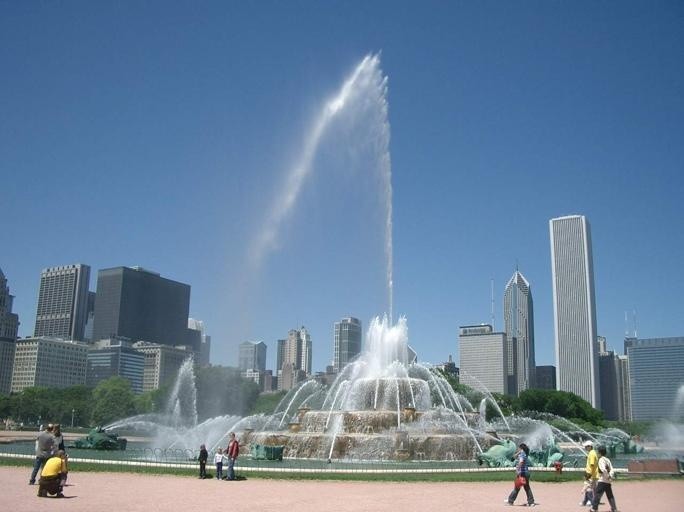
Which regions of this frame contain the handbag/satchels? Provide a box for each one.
[515,477,526,487]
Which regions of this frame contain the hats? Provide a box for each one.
[583,440,592,446]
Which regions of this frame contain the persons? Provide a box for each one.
[222,432,238,479]
[589,445,618,512]
[197,444,207,479]
[504,443,536,506]
[579,473,593,506]
[582,440,605,504]
[213,447,227,479]
[28,423,56,485]
[36,448,66,498]
[52,424,67,486]
